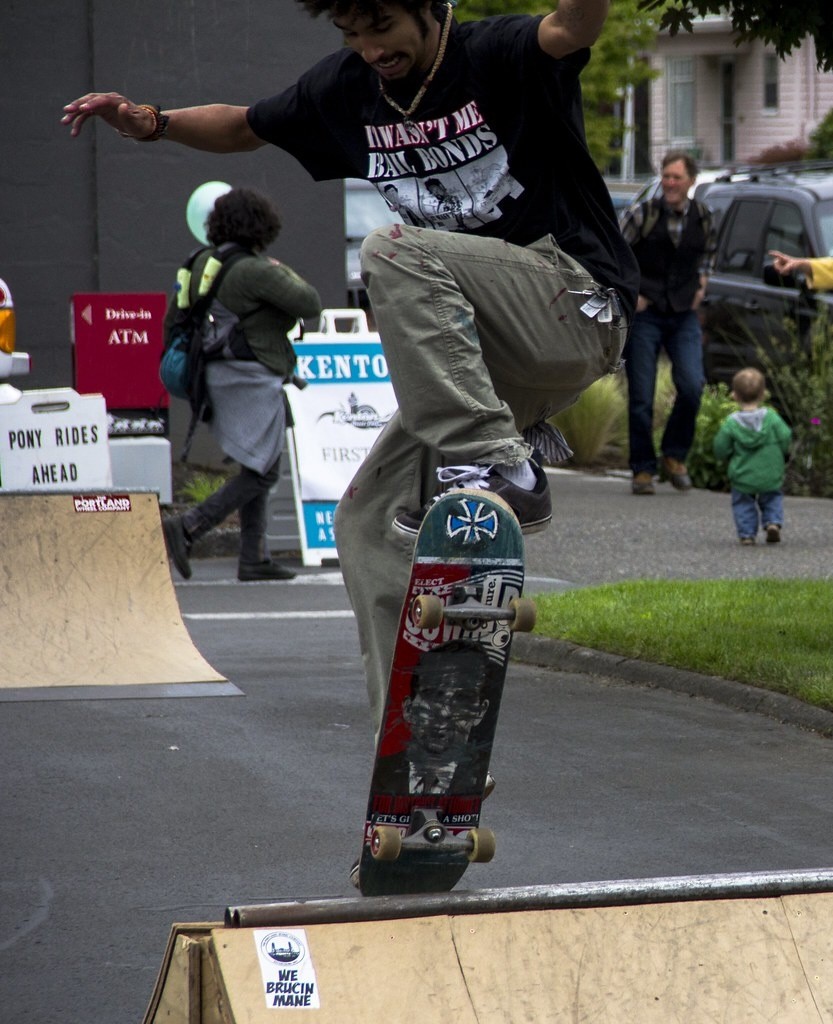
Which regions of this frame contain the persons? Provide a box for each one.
[162,186,322,582]
[768,250,833,290]
[60,0,641,889]
[713,367,792,546]
[618,151,718,494]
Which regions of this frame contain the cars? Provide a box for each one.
[618,160,832,427]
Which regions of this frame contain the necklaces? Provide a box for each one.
[379,2,453,132]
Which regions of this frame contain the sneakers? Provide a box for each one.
[391,457,553,539]
[349,772,495,889]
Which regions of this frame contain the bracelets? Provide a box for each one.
[114,105,170,142]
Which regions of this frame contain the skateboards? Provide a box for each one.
[357,490,539,897]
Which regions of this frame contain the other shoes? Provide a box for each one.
[742,536,755,545]
[237,560,296,581]
[656,456,692,490]
[631,470,655,493]
[765,525,781,542]
[163,515,192,581]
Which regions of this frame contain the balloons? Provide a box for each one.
[186,181,232,246]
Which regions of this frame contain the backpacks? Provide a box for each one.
[159,241,248,424]
